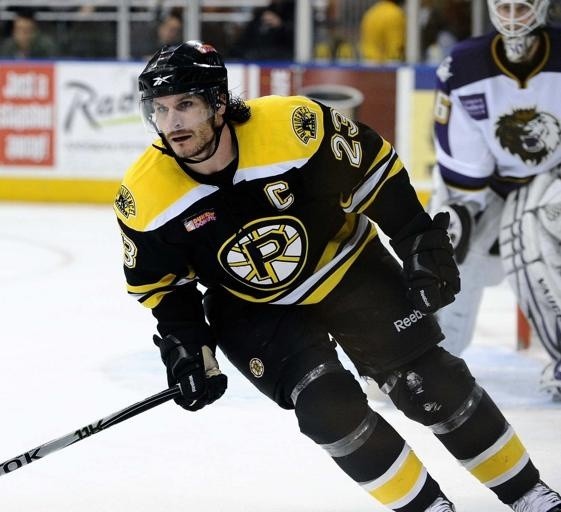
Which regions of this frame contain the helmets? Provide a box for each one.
[138,40,227,132]
[487,0,550,38]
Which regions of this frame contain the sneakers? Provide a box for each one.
[508,478,561,511]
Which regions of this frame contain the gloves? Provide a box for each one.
[389,212,461,314]
[428,204,462,249]
[153,320,227,411]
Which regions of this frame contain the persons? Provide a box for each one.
[114,41,561,512]
[429,1,560,405]
[1,1,495,74]
